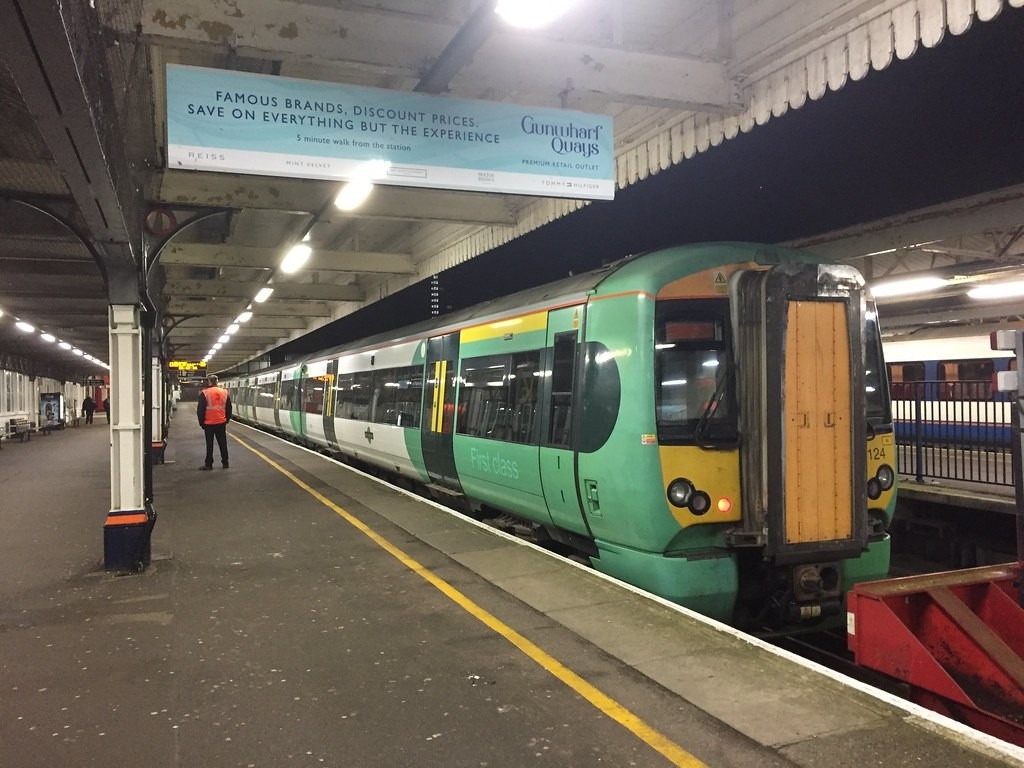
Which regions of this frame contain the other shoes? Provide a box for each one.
[222,464,229,468]
[199,465,213,471]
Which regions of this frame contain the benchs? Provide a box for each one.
[39,416,54,435]
[11,418,33,443]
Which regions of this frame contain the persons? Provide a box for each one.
[442,395,467,434]
[103,394,110,424]
[197,375,232,470]
[83,397,98,424]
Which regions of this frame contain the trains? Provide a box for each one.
[220,242,902,638]
[881,318,1024,499]
